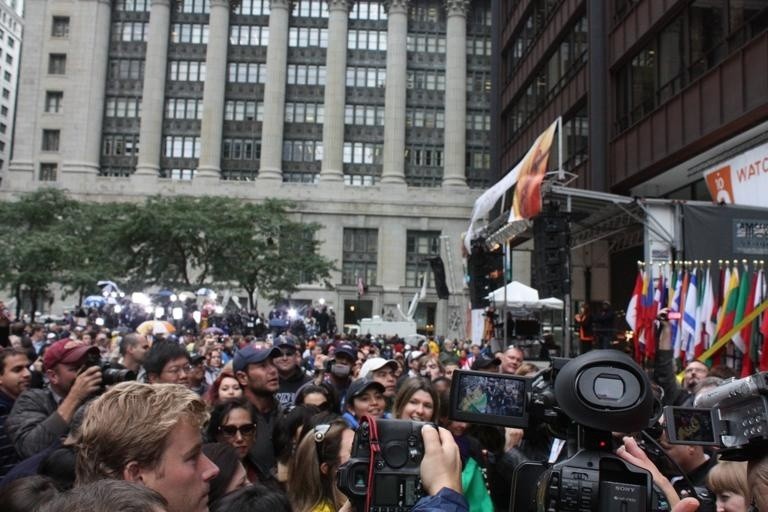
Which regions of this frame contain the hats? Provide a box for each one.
[409,351,425,361]
[232,344,283,371]
[273,335,296,349]
[359,357,399,377]
[474,351,501,369]
[345,377,385,402]
[335,342,357,360]
[43,338,101,370]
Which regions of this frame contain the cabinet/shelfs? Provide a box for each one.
[484,292,571,361]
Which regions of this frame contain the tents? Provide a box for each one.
[540,299,564,309]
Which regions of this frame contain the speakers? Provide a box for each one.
[424,256,449,300]
[529,212,571,300]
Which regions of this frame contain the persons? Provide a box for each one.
[575,301,593,354]
[0,299,539,512]
[594,301,613,349]
[616,413,767,512]
[685,361,736,407]
[539,330,555,361]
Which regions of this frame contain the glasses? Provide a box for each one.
[285,351,294,357]
[218,423,257,438]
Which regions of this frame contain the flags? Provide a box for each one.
[625,264,768,379]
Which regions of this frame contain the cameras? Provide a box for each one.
[83,354,136,396]
[337,418,439,512]
[657,486,717,512]
[322,358,350,376]
[661,311,681,320]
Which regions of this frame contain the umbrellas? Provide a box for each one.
[81,280,217,308]
